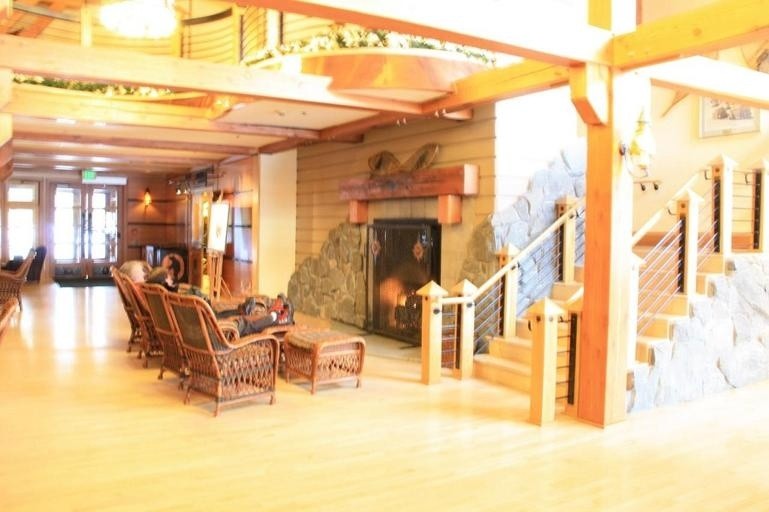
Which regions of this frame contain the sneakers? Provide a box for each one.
[278,304,293,324]
[271,295,285,315]
[239,297,255,315]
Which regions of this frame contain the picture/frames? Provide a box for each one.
[699,94,762,140]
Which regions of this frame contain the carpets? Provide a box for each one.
[56,277,115,287]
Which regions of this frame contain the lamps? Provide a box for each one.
[143,186,152,206]
[96,1,177,40]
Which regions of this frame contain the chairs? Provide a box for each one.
[0,249,38,311]
[108,264,281,419]
[6,246,47,281]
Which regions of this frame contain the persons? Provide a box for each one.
[145,266,295,340]
[143,260,256,320]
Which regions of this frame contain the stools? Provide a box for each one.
[261,322,366,395]
